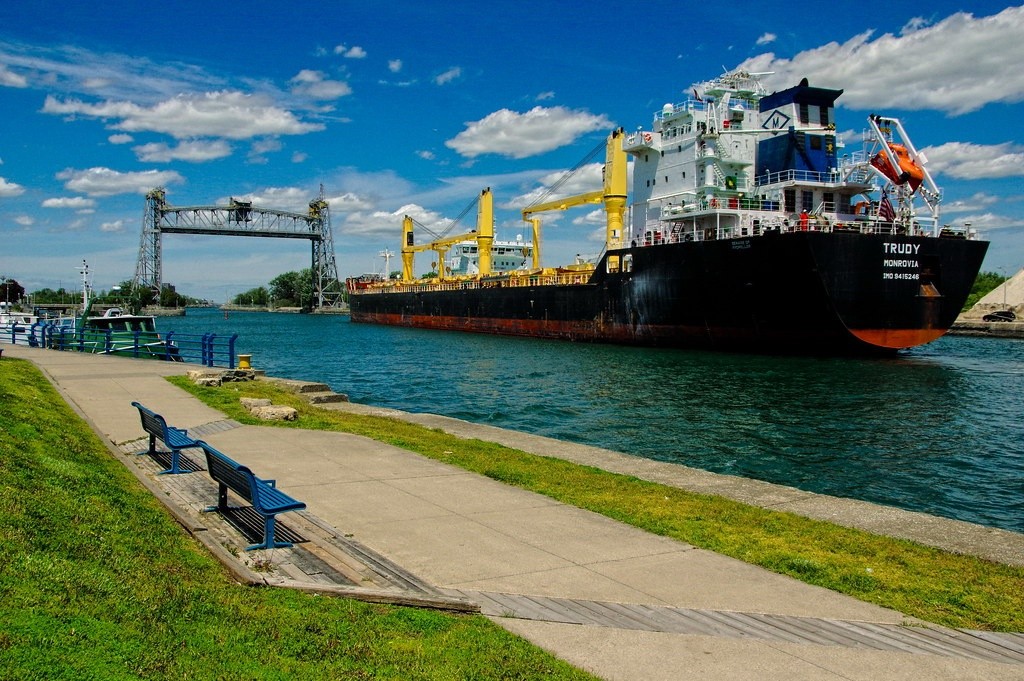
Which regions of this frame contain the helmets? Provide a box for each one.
[803,209,807,212]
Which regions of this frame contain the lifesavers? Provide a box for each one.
[645,132,652,144]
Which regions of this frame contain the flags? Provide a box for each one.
[879,190,896,222]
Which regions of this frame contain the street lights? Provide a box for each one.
[997,266,1006,310]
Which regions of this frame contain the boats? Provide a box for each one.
[0,257,183,361]
[344,65,992,352]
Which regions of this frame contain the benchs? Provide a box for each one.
[130,401,199,474]
[194,439,307,551]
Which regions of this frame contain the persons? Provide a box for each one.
[799,209,809,231]
[859,202,867,215]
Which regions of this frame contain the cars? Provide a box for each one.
[982,310,1016,322]
